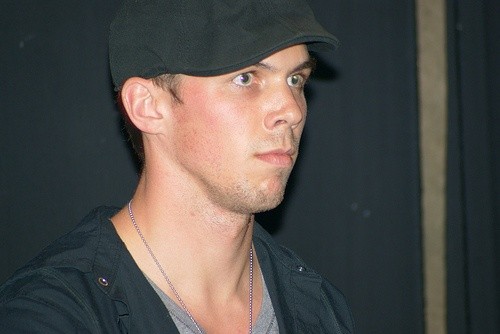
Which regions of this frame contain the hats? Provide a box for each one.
[108,0,342,92]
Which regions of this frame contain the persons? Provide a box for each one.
[0,0,355,334]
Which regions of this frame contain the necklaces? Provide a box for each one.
[128,198,253,334]
